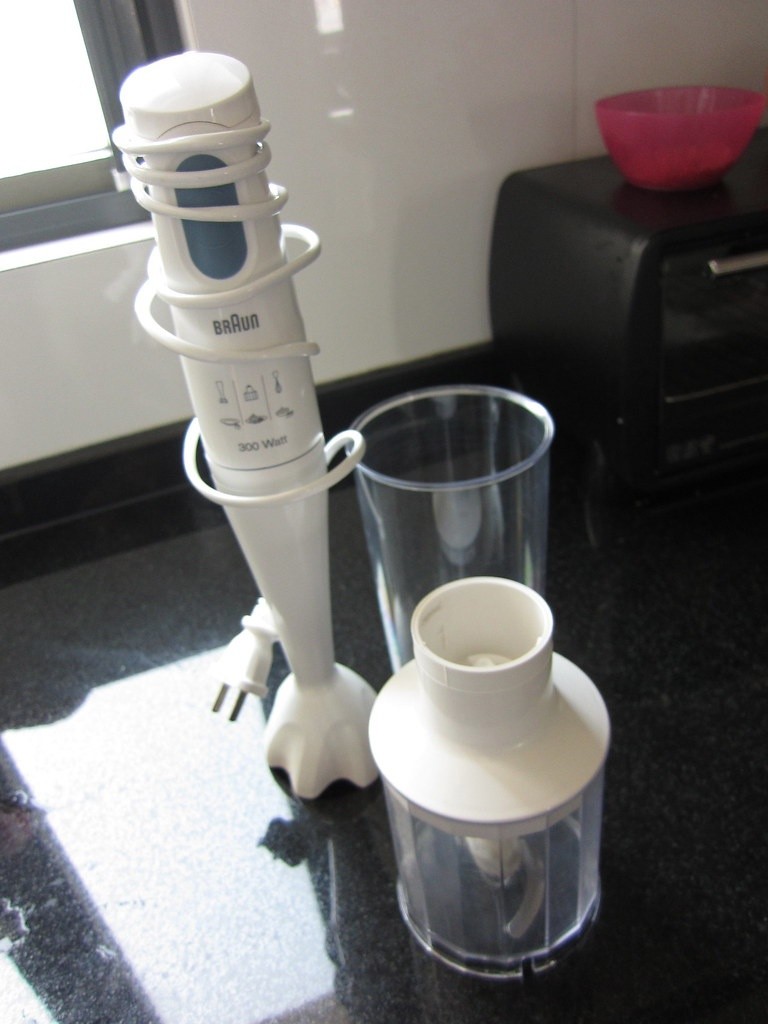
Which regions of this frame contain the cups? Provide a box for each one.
[344,386,555,676]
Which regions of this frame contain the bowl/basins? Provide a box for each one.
[595,86,767,193]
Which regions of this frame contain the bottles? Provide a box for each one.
[368,575,612,980]
